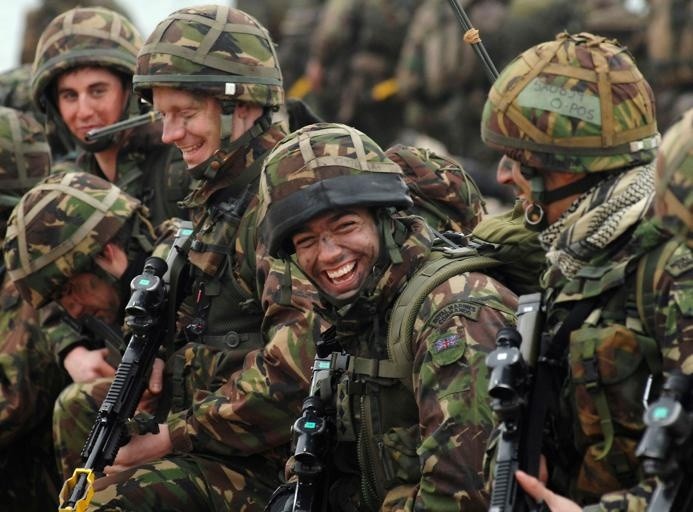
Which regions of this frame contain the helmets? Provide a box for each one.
[1,106,53,206]
[480,34,662,175]
[33,7,142,113]
[259,122,414,257]
[134,6,286,108]
[2,169,142,311]
[0,64,35,111]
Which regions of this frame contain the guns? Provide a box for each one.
[284,344,335,511]
[637,373,690,512]
[487,291,577,512]
[54,222,206,511]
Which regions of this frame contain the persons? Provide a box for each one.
[1,0,692,512]
